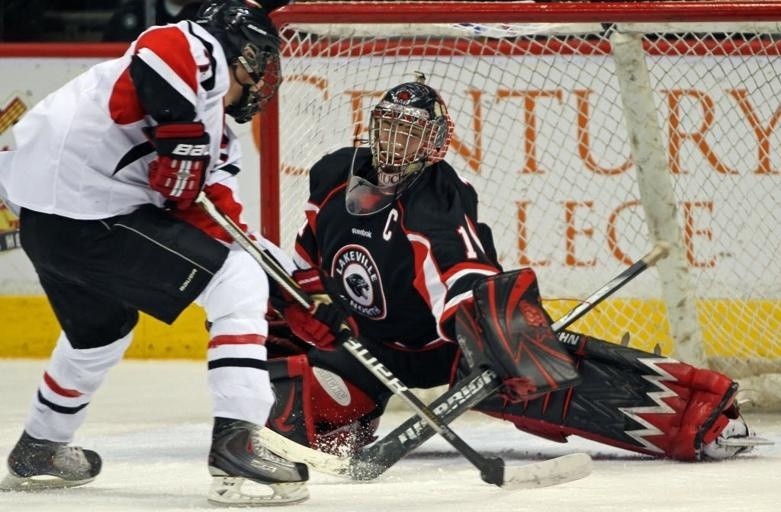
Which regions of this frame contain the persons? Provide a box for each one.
[0,0,309,484]
[266,82,752,459]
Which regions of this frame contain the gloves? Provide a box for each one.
[271,268,359,351]
[142,123,210,212]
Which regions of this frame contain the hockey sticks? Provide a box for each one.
[251,241,669,482]
[196,191,595,489]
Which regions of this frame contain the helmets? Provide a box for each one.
[198,0,281,83]
[369,82,454,149]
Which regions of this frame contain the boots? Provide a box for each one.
[208,416,309,484]
[7,431,101,479]
[704,414,753,458]
